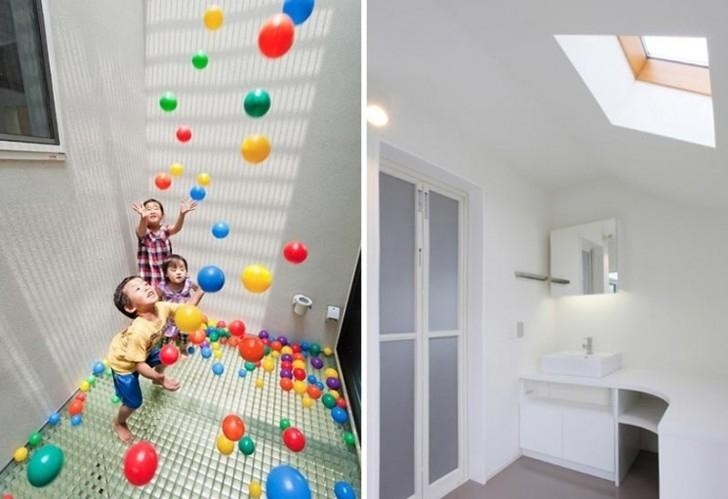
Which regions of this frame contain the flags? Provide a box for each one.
[159,303,356,499]
[159,95,177,111]
[176,126,192,142]
[243,89,271,117]
[258,0,315,58]
[0,358,158,499]
[241,263,273,293]
[190,186,206,201]
[240,134,271,164]
[197,265,225,294]
[202,7,223,31]
[282,240,308,263]
[196,172,211,187]
[169,163,184,177]
[155,174,171,190]
[211,220,229,238]
[191,52,208,69]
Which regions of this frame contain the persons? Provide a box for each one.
[108,275,205,443]
[132,198,198,290]
[151,254,198,385]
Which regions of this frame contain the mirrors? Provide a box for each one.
[551,218,620,298]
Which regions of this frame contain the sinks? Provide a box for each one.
[544,349,624,377]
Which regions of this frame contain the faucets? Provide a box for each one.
[581,335,594,352]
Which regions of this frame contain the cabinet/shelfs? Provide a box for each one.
[616,388,728,499]
[518,376,617,486]
